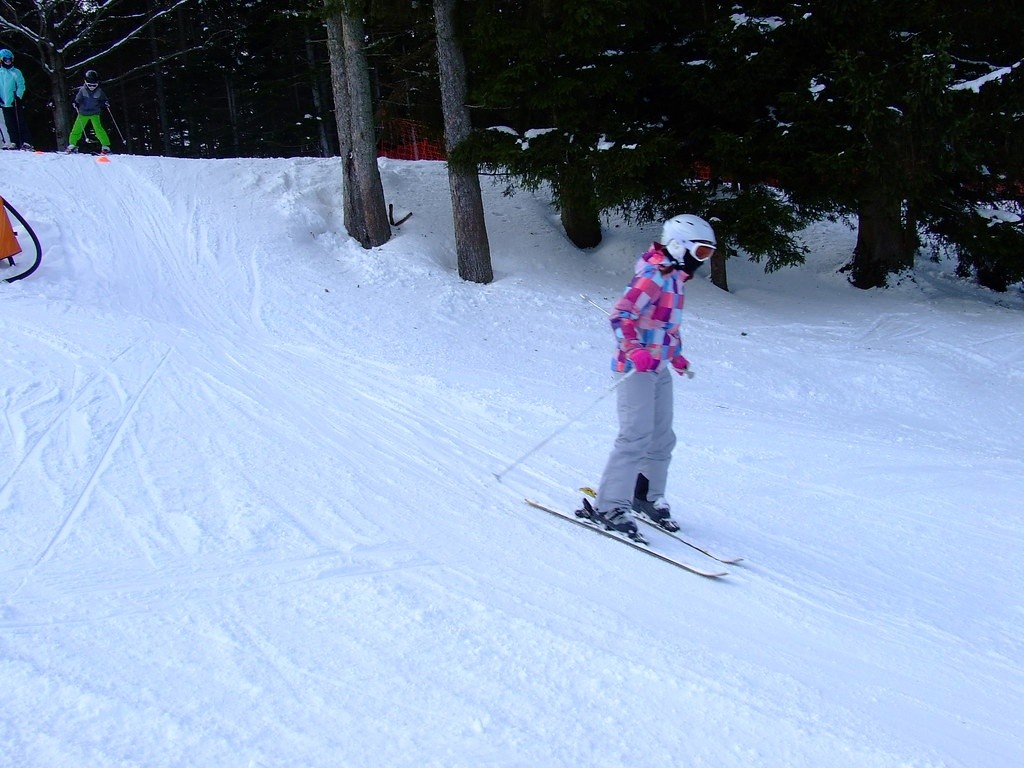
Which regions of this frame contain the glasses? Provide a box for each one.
[690,241,717,262]
[87,83,98,88]
[2,57,13,62]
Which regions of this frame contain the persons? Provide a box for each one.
[66,69,112,153]
[590,212,714,529]
[0,49,34,149]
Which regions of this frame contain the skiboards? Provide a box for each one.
[524,487,746,580]
[54,150,113,156]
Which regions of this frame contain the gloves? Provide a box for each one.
[11,97,20,106]
[671,355,688,375]
[631,349,653,373]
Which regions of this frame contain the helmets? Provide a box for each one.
[661,214,716,262]
[85,70,99,83]
[0,49,14,61]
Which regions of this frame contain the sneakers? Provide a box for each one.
[634,493,671,524]
[597,506,638,532]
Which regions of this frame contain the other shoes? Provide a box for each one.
[65,145,77,153]
[102,146,110,153]
